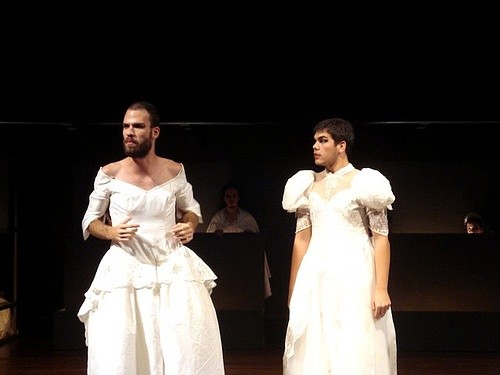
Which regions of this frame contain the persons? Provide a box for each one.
[464,213,485,233]
[206,185,273,299]
[282,118,398,375]
[76,102,226,375]
